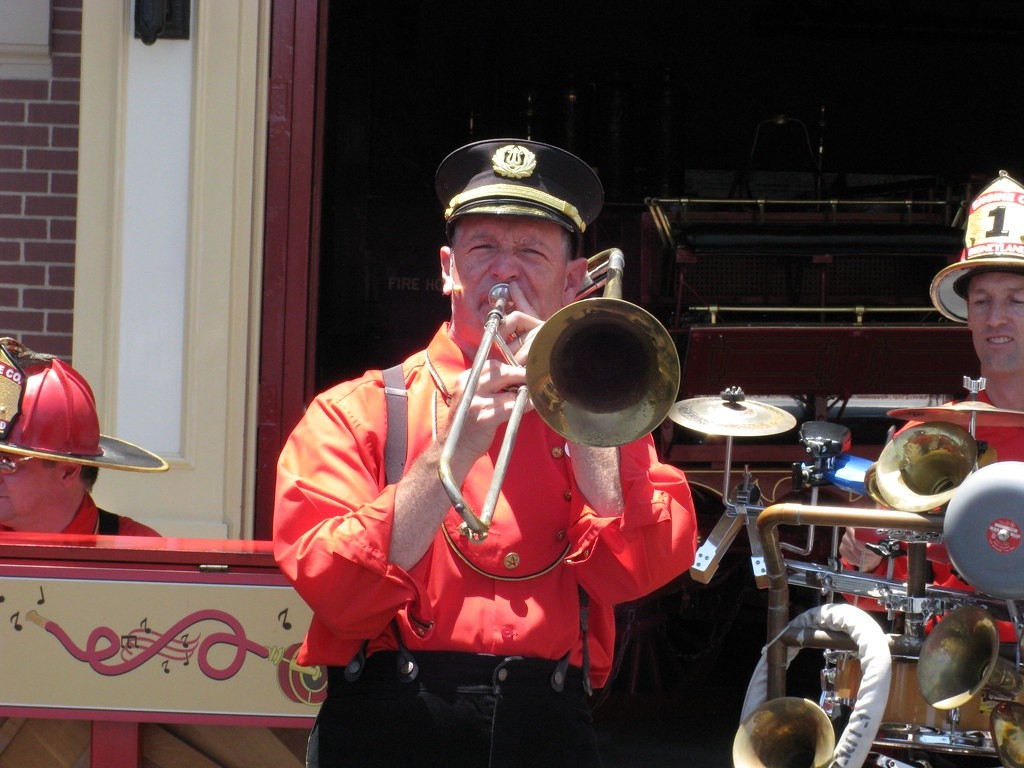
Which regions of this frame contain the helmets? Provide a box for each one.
[929,169,1024,323]
[0,336,169,472]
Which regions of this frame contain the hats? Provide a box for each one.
[435,138,604,248]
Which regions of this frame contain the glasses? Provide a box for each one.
[0,457,33,474]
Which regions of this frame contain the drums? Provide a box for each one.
[830,648,1024,757]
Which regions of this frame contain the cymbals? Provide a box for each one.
[886,373,1024,429]
[666,383,799,439]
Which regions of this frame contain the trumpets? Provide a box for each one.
[435,243,689,546]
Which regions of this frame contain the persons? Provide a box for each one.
[272,138,698,768]
[839,169,1024,644]
[1,336,169,538]
